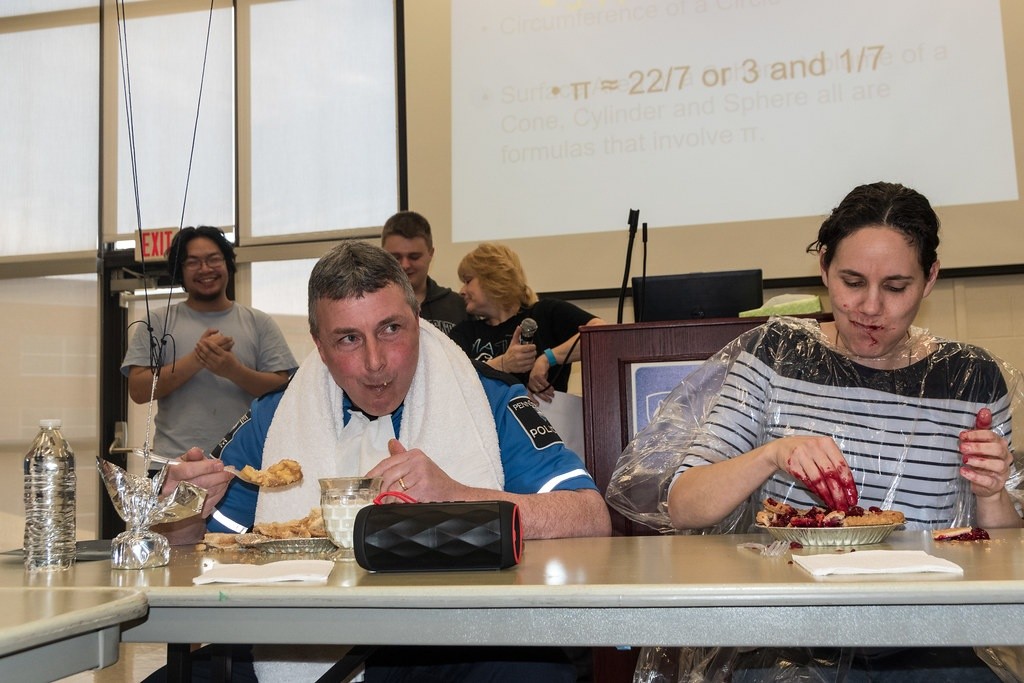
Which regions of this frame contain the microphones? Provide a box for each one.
[519,319,538,345]
[639,223,648,323]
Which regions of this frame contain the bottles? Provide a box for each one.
[23,418,77,575]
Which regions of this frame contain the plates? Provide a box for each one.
[235,532,337,553]
[755,521,906,546]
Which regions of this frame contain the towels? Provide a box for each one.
[255,315,503,533]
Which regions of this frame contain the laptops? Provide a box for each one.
[632,268,764,324]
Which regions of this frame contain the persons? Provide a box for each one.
[448,244,604,407]
[121,226,300,477]
[382,213,468,334]
[162,240,613,683]
[605,181,1024,683]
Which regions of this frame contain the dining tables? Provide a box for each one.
[0,526,1024,683]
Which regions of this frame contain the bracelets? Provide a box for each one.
[545,348,557,367]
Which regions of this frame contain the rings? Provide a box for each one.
[531,387,537,392]
[536,383,542,389]
[399,478,408,491]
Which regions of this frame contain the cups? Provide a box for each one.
[318,477,385,559]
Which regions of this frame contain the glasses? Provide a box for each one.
[178,256,228,271]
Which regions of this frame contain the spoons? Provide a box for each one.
[133,448,303,488]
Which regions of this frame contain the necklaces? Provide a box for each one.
[836,330,912,366]
